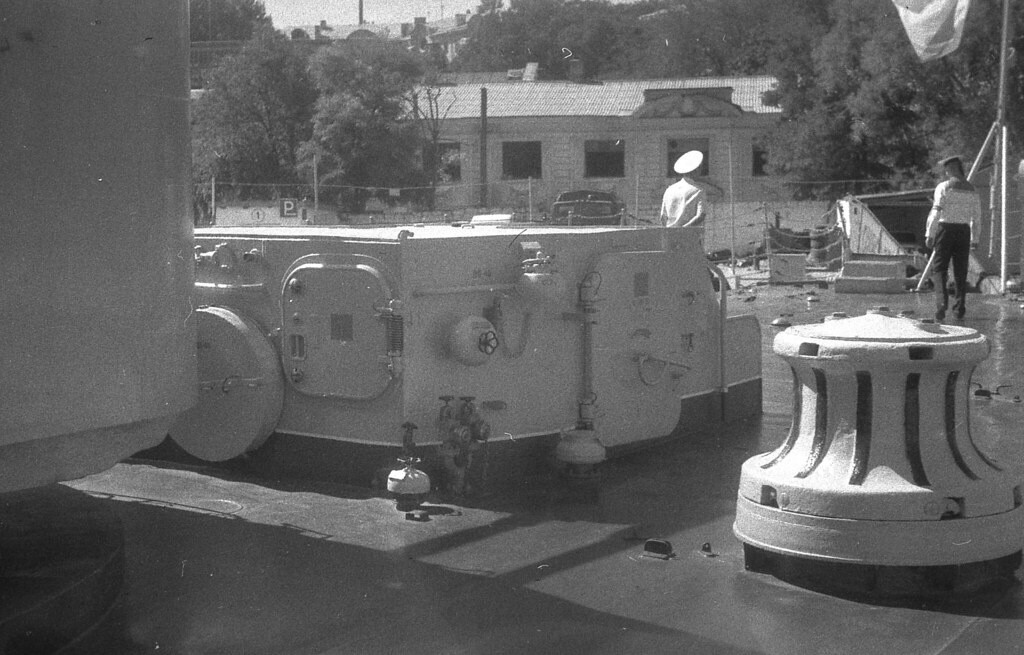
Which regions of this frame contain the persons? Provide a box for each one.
[924,156,981,322]
[659,151,707,227]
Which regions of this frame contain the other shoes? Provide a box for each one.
[951,311,965,320]
[934,312,945,319]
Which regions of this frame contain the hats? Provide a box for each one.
[673,149,704,174]
[938,156,959,164]
